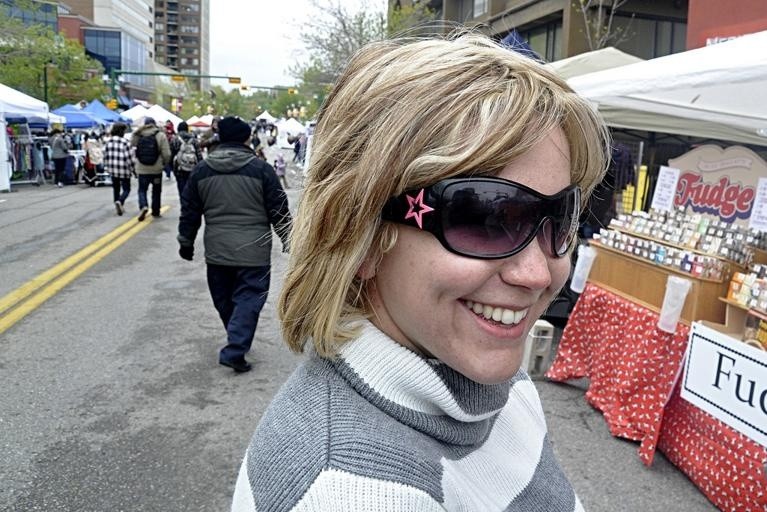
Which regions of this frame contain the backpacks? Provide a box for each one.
[135,132,158,166]
[175,135,198,171]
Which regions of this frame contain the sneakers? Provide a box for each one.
[219,357,252,373]
[116,200,124,215]
[138,207,148,221]
[58,183,63,188]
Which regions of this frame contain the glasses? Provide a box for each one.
[381,174,580,260]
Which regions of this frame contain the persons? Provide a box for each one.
[177,114,295,373]
[233,23,612,510]
[156,114,308,199]
[609,135,636,194]
[102,122,138,217]
[45,129,71,188]
[62,126,111,189]
[130,116,173,221]
[576,171,614,239]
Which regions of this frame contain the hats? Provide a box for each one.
[178,121,188,132]
[219,117,251,144]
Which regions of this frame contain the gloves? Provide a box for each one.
[179,246,195,261]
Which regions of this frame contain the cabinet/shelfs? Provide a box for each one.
[585,224,767,353]
